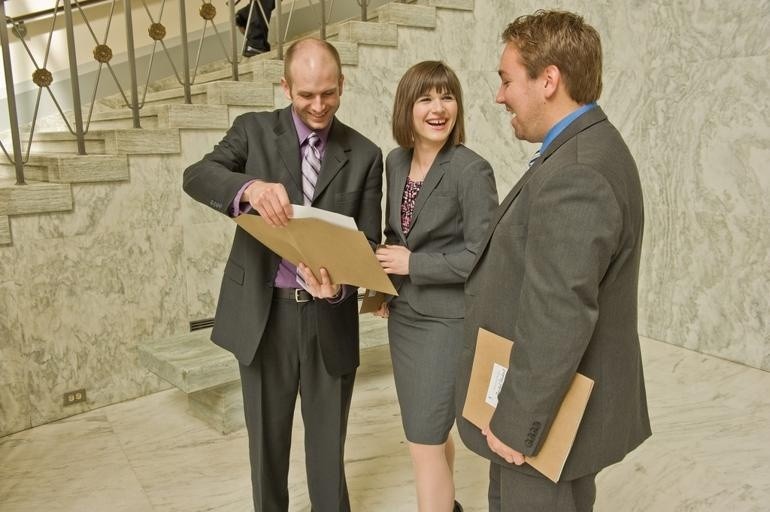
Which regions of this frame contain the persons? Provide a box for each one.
[373,59,501,511]
[227,1,283,57]
[179,35,384,512]
[448,7,654,511]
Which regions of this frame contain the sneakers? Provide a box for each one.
[243,41,269,57]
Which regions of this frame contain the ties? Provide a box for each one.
[302,132,322,207]
[529,150,542,167]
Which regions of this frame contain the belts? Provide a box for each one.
[273,286,311,301]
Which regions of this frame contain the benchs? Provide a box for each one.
[135,299,388,434]
